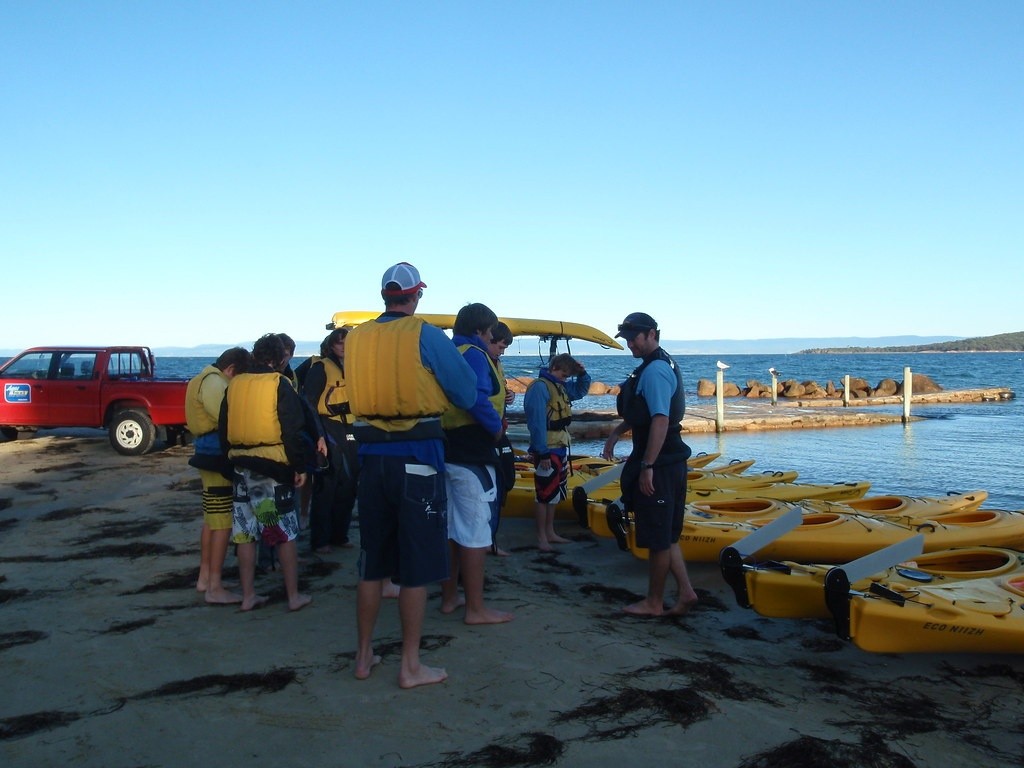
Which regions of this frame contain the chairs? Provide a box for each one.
[56,363,80,379]
[78,361,94,380]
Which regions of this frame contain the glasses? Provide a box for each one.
[618,323,650,331]
[418,288,423,298]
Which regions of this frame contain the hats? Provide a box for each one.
[614,313,657,340]
[382,262,427,296]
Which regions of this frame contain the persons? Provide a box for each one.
[523,353,592,553]
[602,312,700,617]
[184,262,517,689]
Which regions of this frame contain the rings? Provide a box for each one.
[543,465,545,466]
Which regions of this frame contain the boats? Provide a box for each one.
[494,447,1023,661]
[324,309,625,353]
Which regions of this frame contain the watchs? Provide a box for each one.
[640,461,654,469]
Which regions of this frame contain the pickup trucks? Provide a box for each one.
[0,344,194,457]
